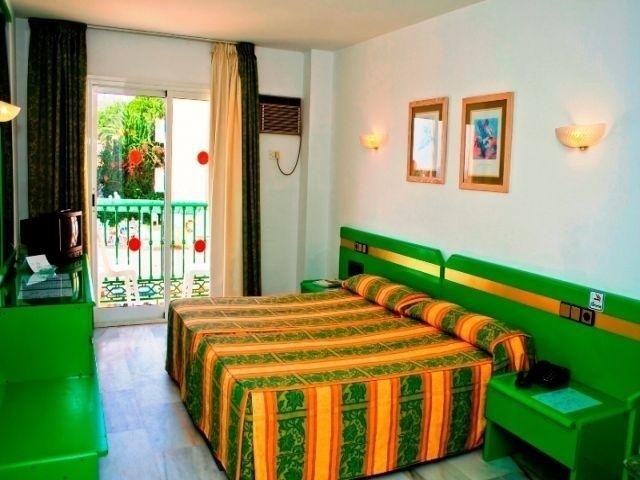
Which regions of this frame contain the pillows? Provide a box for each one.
[342,274,531,360]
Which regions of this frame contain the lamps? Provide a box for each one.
[556,123,606,151]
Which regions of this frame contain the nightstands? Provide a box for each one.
[482,370,629,479]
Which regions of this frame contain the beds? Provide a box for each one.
[172,292,493,479]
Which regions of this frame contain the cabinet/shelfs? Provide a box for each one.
[1,254,109,479]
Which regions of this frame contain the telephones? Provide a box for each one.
[529,360,570,388]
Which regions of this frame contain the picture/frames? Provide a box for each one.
[405,91,513,193]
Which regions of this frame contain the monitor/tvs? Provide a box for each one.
[19,210,88,267]
[21,269,88,305]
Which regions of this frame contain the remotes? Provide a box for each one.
[57,208,71,212]
